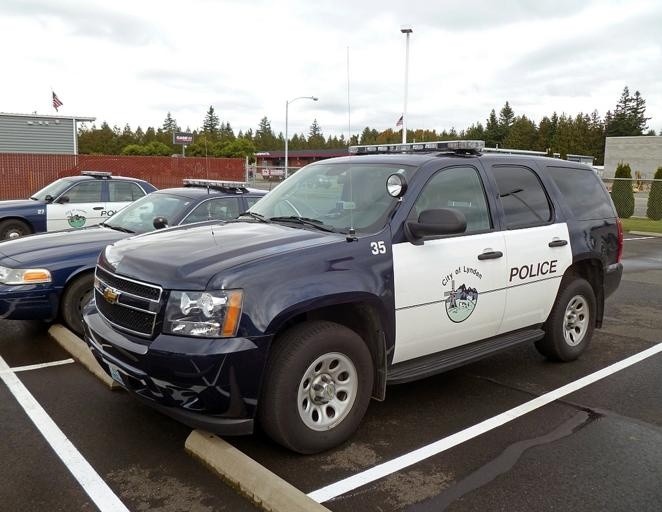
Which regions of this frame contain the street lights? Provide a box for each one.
[285,95,320,183]
[400,26,414,152]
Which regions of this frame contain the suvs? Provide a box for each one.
[83,134,624,455]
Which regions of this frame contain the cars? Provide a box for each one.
[1,178,331,334]
[1,170,160,242]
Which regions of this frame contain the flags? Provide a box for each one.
[52,91,64,108]
